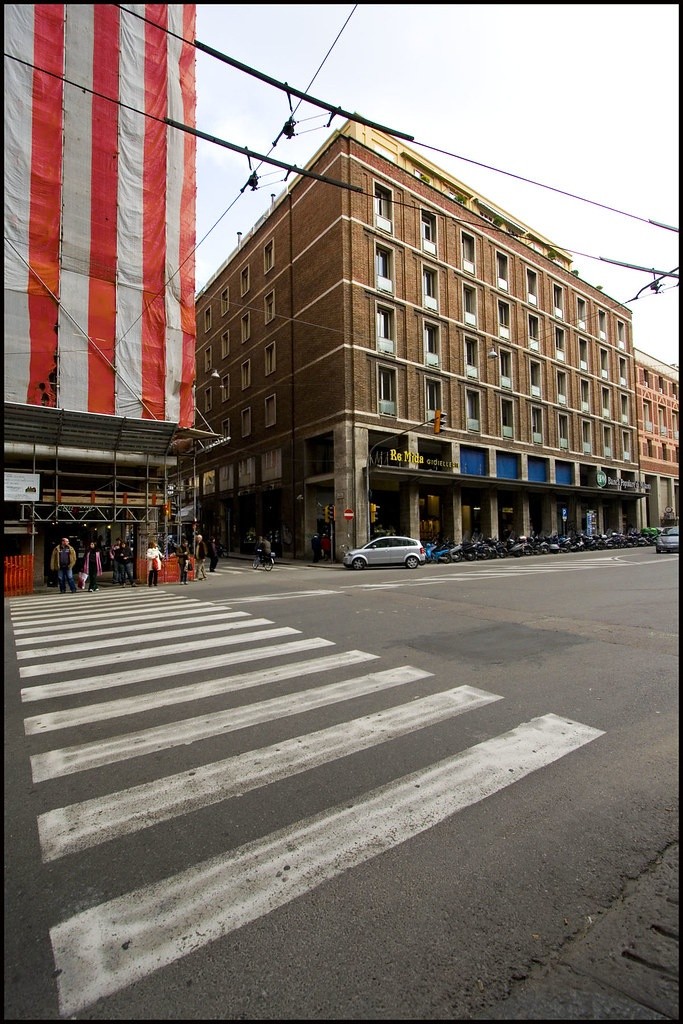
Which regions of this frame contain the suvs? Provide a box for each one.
[342,536,426,569]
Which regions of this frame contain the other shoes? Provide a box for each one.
[72,588,76,593]
[61,590,65,593]
[149,584,152,587]
[88,588,93,592]
[184,581,188,584]
[95,588,99,591]
[154,584,158,586]
[131,582,140,587]
[201,577,208,581]
[180,581,184,585]
[122,582,126,588]
[193,578,198,581]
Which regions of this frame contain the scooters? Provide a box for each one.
[252,549,276,571]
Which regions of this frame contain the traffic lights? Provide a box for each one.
[322,506,330,523]
[370,503,380,524]
[327,505,336,522]
[434,410,448,434]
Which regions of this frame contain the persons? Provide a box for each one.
[82,542,103,592]
[311,533,331,563]
[255,536,271,567]
[176,540,190,585]
[109,537,139,588]
[50,538,77,594]
[208,536,219,572]
[146,541,164,587]
[194,534,207,582]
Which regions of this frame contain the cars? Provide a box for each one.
[656,526,679,553]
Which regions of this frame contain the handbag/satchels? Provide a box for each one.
[152,558,158,571]
[78,572,89,590]
[188,564,192,571]
[184,560,190,572]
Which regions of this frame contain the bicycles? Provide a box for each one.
[420,527,665,565]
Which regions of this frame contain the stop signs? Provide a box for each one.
[343,508,354,521]
